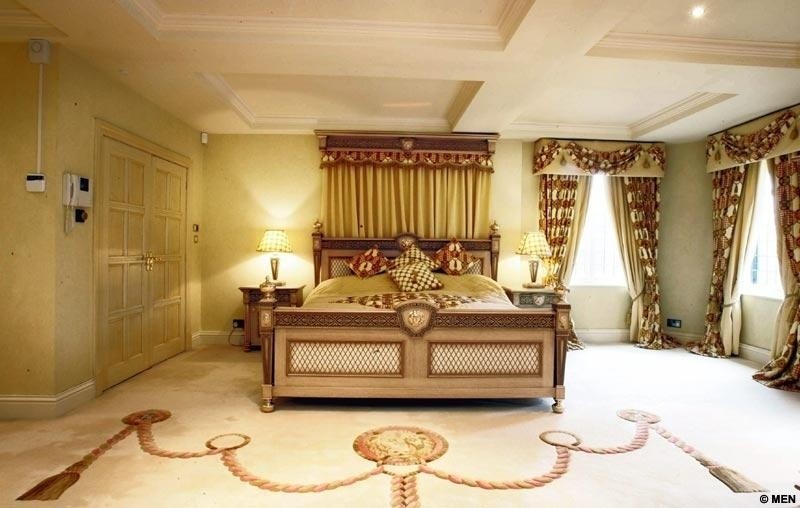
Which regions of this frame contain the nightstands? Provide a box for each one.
[238,284,308,353]
[503,283,559,310]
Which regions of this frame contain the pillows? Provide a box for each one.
[343,236,481,293]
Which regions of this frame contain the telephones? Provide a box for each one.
[62,173,93,208]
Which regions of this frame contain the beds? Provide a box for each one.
[256,219,572,413]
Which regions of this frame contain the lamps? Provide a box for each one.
[257,230,296,286]
[515,229,554,288]
